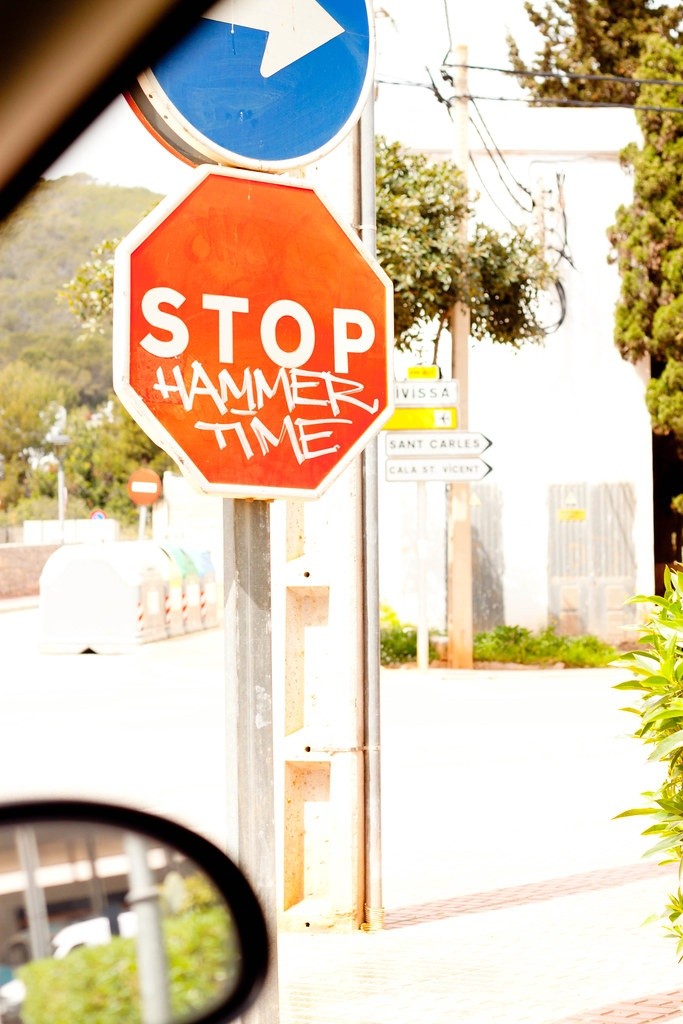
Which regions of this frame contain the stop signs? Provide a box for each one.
[110,165,397,498]
[125,469,162,505]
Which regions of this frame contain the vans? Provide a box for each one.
[0,909,139,1024]
